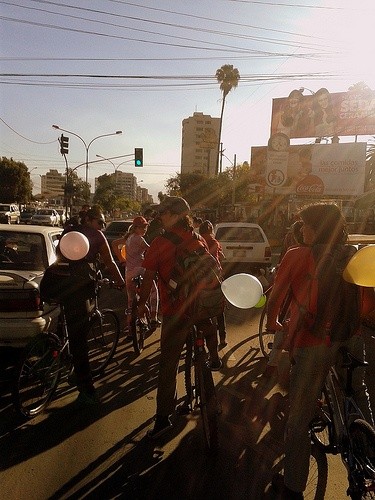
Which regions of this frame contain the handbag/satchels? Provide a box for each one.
[39,263,98,306]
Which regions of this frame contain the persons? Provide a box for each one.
[272,88,336,138]
[248,202,375,500]
[55,196,227,445]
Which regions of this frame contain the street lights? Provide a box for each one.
[96,154,135,221]
[52,125,122,185]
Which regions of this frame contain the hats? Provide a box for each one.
[150,196,190,217]
[133,217,149,227]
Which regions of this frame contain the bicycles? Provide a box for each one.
[183,256,225,466]
[9,276,125,419]
[258,265,375,500]
[132,272,158,354]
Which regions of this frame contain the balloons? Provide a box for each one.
[120,245,126,260]
[255,294,266,308]
[220,273,263,309]
[59,231,90,260]
[342,245,375,287]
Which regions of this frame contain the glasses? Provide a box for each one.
[90,216,106,228]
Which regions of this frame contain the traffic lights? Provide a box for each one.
[135,148,143,167]
[60,134,69,155]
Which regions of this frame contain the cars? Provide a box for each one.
[0,203,69,227]
[103,220,133,261]
[213,223,273,273]
[0,223,65,348]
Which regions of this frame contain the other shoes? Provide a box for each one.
[148,419,172,439]
[271,474,304,500]
[127,335,134,340]
[150,319,162,328]
[78,393,95,405]
[207,357,222,371]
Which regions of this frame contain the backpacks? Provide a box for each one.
[158,232,223,321]
[292,242,360,343]
